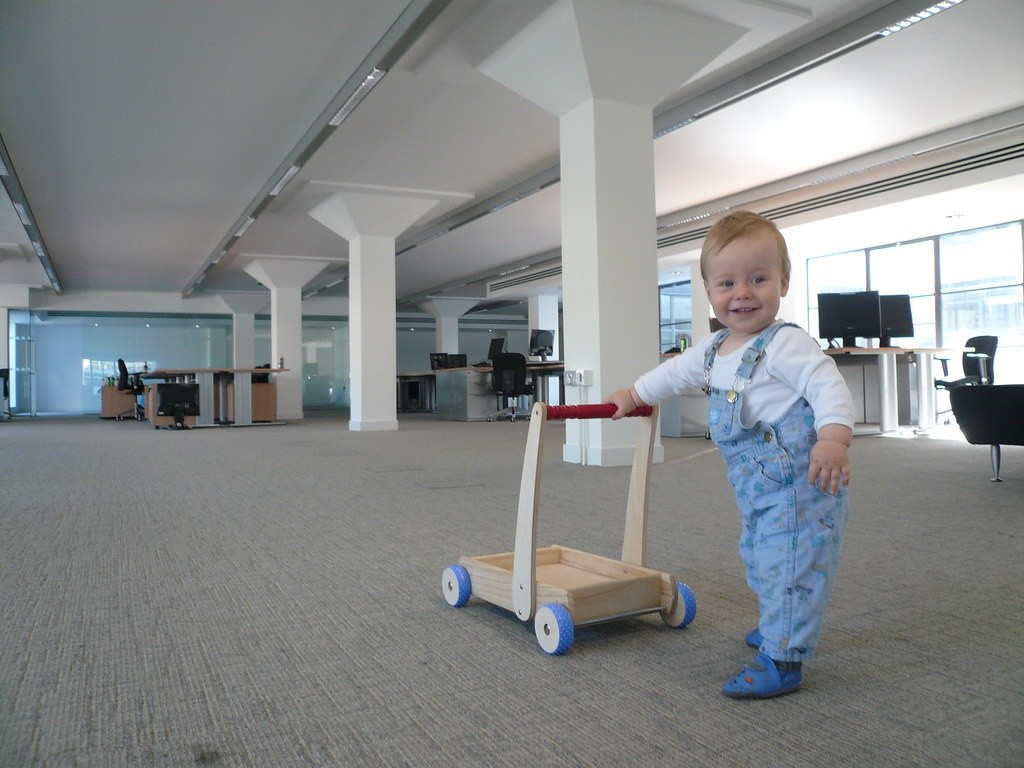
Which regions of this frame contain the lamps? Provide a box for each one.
[0,136,65,296]
[181,0,451,299]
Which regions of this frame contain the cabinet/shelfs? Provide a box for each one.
[99,385,136,418]
[226,383,276,422]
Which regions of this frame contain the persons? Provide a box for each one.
[602,211,857,701]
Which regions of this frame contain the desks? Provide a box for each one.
[657,353,708,438]
[396,371,436,412]
[140,368,292,429]
[822,347,975,435]
[434,364,564,422]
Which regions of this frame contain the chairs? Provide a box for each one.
[115,359,152,420]
[929,335,999,425]
[487,352,534,422]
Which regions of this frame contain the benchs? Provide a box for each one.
[950,384,1024,483]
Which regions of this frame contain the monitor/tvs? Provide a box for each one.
[528,329,554,362]
[878,295,914,348]
[818,291,882,347]
[488,338,504,360]
[430,353,468,371]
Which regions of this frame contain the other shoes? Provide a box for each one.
[744,627,807,653]
[723,654,802,699]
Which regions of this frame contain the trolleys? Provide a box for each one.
[441,402,699,655]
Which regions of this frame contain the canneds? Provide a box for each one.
[680,337,686,352]
[106,377,114,386]
[279,358,284,368]
